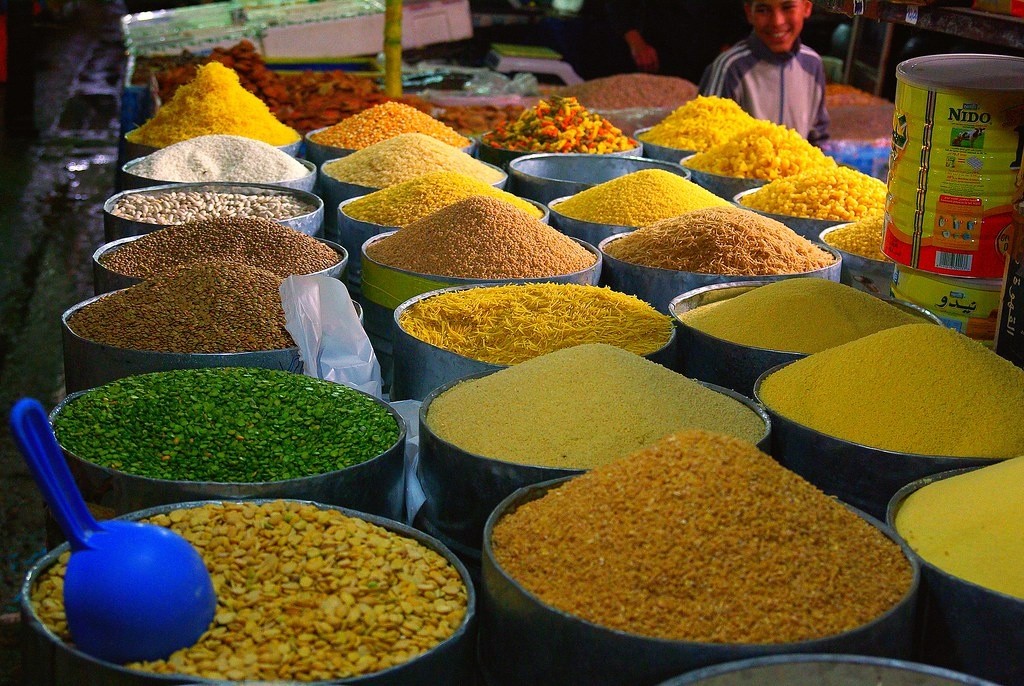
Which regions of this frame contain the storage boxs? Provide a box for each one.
[123,0,472,57]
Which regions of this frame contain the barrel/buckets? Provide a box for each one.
[885,467,1024,686]
[478,126,713,154]
[754,361,1016,522]
[60,289,363,396]
[732,187,854,243]
[417,370,1003,686]
[545,182,843,318]
[305,125,476,166]
[337,194,549,277]
[818,222,894,299]
[546,195,637,246]
[94,186,348,296]
[361,231,675,400]
[679,155,768,201]
[47,383,406,554]
[509,153,691,206]
[322,158,508,216]
[121,131,318,190]
[23,498,476,686]
[668,282,946,403]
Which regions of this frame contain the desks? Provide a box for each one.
[874,0,1024,97]
[813,0,878,84]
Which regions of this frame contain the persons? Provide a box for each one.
[576,0,751,83]
[694,0,830,155]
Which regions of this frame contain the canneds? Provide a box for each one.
[880,53,1024,368]
